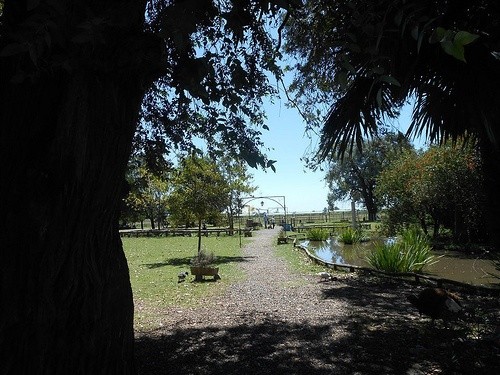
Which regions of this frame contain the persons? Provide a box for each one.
[263,214,268,228]
[271,216,276,228]
[282,216,286,223]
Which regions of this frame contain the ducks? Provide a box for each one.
[178,271,189,284]
[315,271,333,282]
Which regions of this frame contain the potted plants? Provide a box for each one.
[277,229,288,245]
[191,249,221,283]
